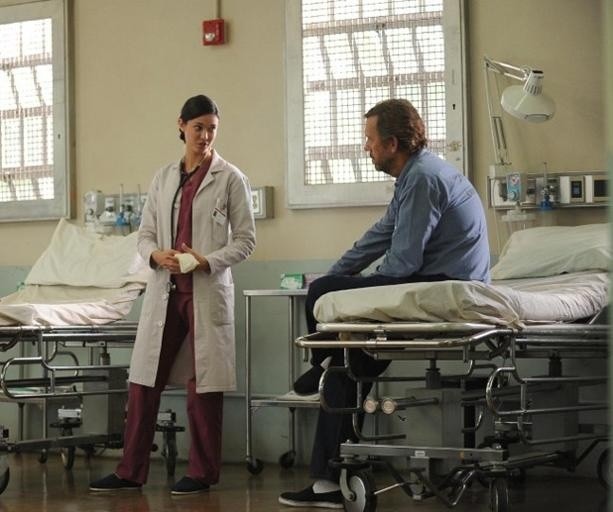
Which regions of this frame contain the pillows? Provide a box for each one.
[488,223,610,279]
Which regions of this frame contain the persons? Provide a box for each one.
[276,95,492,509]
[87,94,258,496]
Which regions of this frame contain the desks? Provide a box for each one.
[242,288,309,471]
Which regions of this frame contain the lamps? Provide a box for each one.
[482,56,556,179]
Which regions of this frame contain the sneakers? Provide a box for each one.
[88,474,143,492]
[293,365,325,396]
[170,476,210,495]
[279,483,346,509]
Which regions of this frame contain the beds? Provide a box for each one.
[1,219,187,493]
[294,222,611,510]
